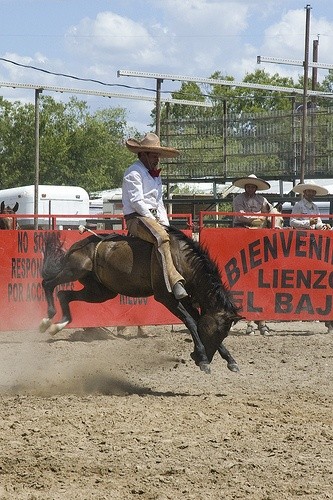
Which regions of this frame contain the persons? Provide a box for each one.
[121,133,188,299]
[290,181,329,230]
[232,174,274,227]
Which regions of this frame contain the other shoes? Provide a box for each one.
[173,283,188,300]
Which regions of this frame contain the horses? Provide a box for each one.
[243,204,284,335]
[39,224,241,373]
[0,200,20,230]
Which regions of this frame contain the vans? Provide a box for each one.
[0,185,90,230]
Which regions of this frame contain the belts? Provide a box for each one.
[124,209,157,220]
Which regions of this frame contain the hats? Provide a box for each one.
[292,184,330,196]
[232,174,270,190]
[126,133,180,158]
